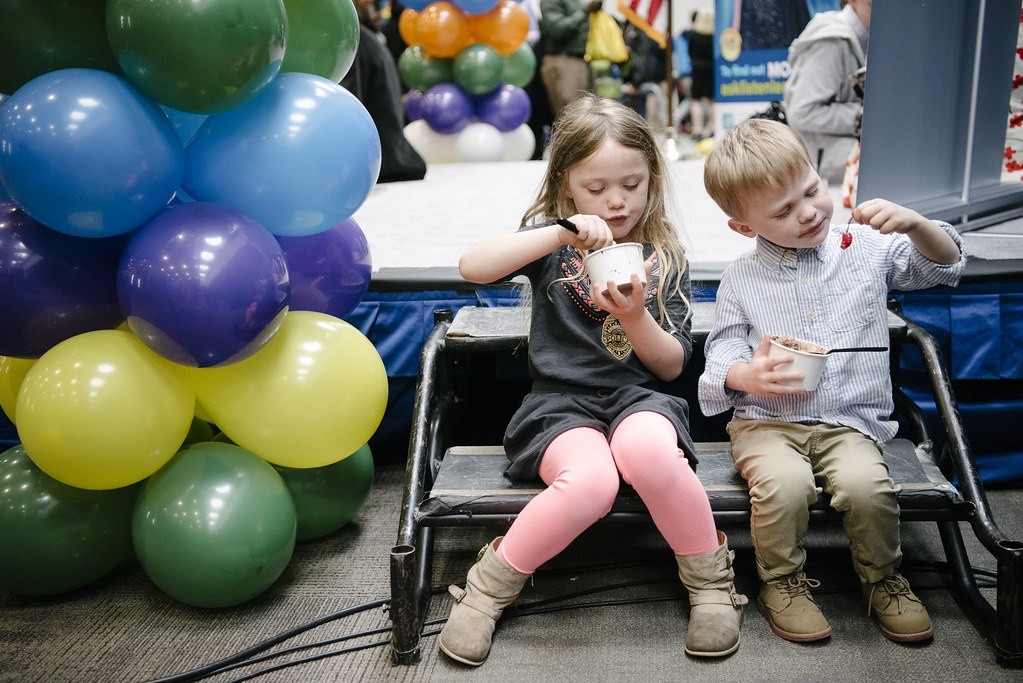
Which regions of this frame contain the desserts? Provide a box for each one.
[774,336,825,356]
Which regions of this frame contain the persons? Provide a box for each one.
[778,0,867,229]
[695,116,970,644]
[379,0,408,127]
[437,95,751,669]
[344,0,430,182]
[526,1,716,141]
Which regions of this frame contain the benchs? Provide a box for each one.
[388,291,1022,673]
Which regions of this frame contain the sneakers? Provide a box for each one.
[758,572,832,641]
[863,571,934,641]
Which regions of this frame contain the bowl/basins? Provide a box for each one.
[769,334,832,392]
[582,242,647,298]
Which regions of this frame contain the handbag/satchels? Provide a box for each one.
[584,6,629,63]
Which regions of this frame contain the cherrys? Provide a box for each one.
[840,217,854,248]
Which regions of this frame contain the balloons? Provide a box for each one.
[1,0,389,612]
[396,0,538,163]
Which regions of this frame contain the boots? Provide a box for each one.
[675,531,748,656]
[438,536,533,665]
